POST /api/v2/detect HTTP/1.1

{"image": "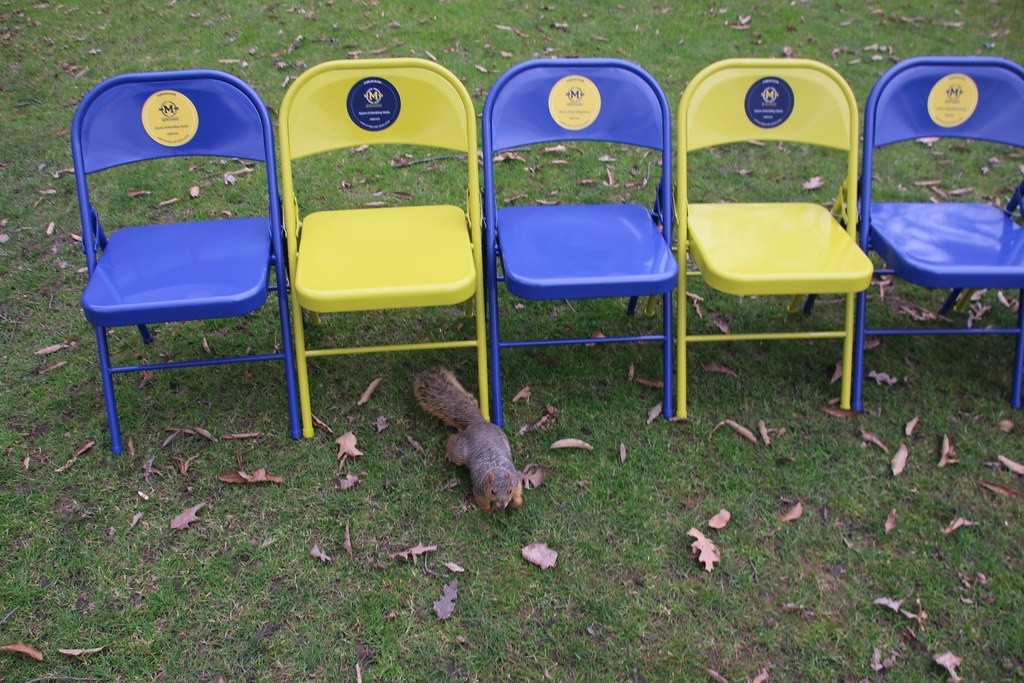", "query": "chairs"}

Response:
[71,70,299,453]
[278,56,492,440]
[670,58,874,420]
[479,57,680,430]
[803,55,1024,412]
